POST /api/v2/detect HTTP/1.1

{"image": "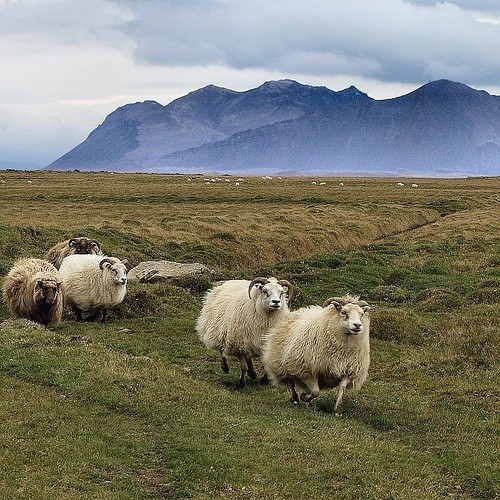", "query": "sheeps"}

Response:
[46,237,101,271]
[262,295,371,418]
[195,276,293,388]
[58,254,129,323]
[3,257,62,323]
